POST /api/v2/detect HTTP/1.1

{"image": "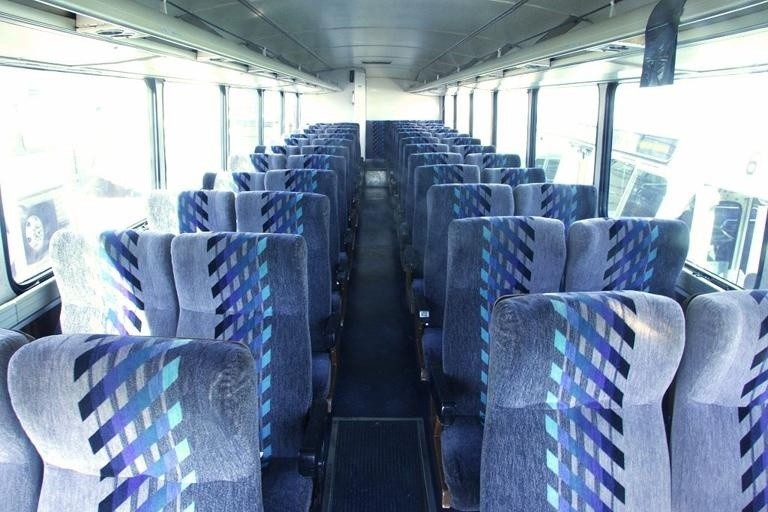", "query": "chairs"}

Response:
[48,227,332,512]
[479,289,686,512]
[671,289,768,512]
[0,327,43,512]
[148,121,363,416]
[430,215,565,511]
[389,120,597,381]
[5,334,265,512]
[563,217,690,298]
[365,120,392,173]
